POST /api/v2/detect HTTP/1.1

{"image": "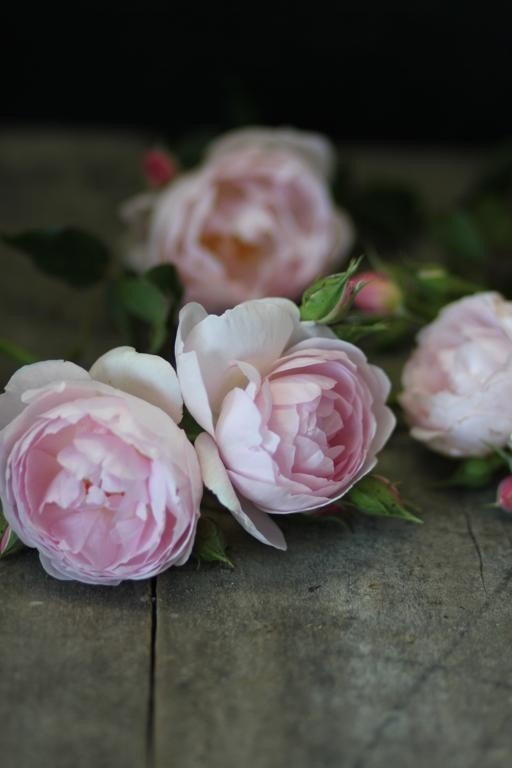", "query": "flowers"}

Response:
[0,115,510,595]
